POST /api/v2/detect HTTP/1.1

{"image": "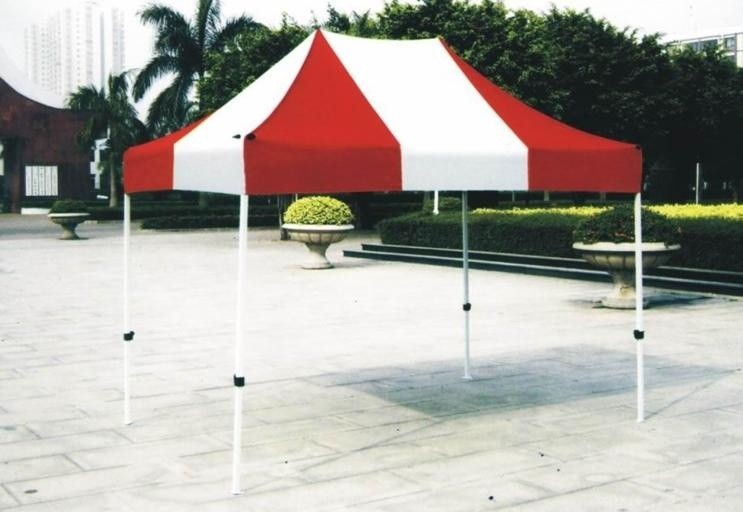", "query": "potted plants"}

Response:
[571,204,683,312]
[279,196,354,270]
[48,200,90,240]
